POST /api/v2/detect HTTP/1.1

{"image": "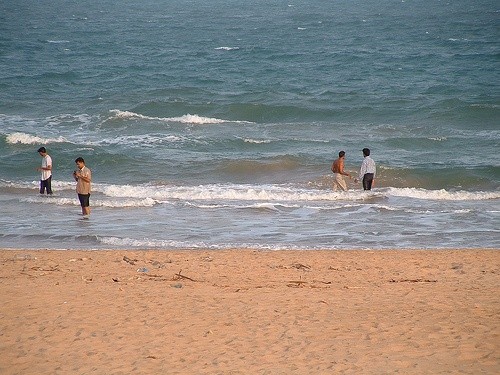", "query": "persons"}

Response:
[73,157,92,216]
[331,151,351,191]
[354,148,377,191]
[37,147,53,195]
